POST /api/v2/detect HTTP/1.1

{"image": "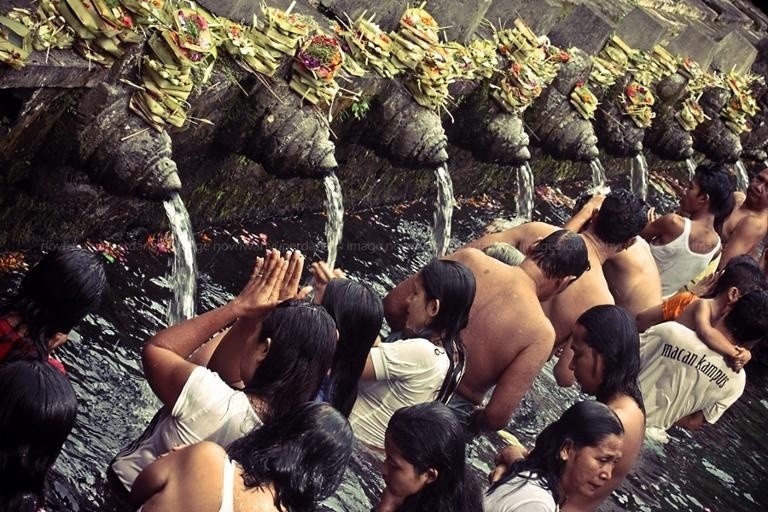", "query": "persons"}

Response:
[110,228,646,509]
[465,166,768,424]
[0,360,100,511]
[0,248,109,375]
